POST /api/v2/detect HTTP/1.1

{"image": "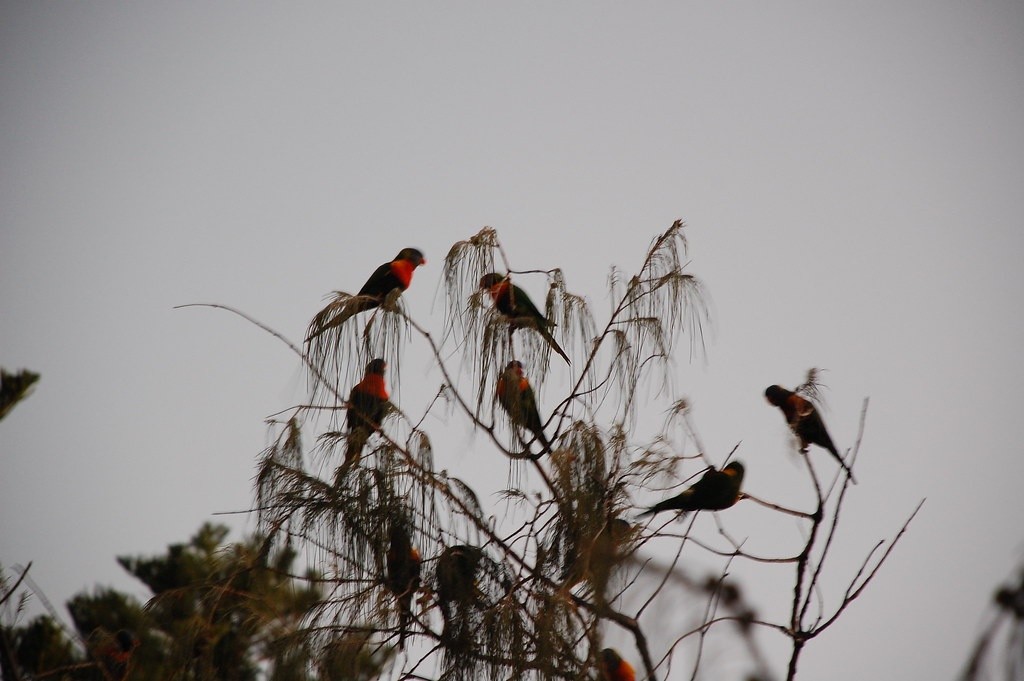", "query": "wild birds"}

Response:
[764,384,859,486]
[595,648,636,681]
[331,358,390,495]
[384,526,423,656]
[477,272,573,368]
[634,461,745,521]
[494,360,556,457]
[301,248,428,344]
